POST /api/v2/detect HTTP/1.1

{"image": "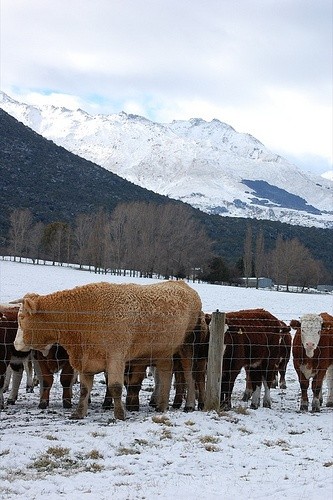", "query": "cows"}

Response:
[289,311,332,413]
[0,279,292,421]
